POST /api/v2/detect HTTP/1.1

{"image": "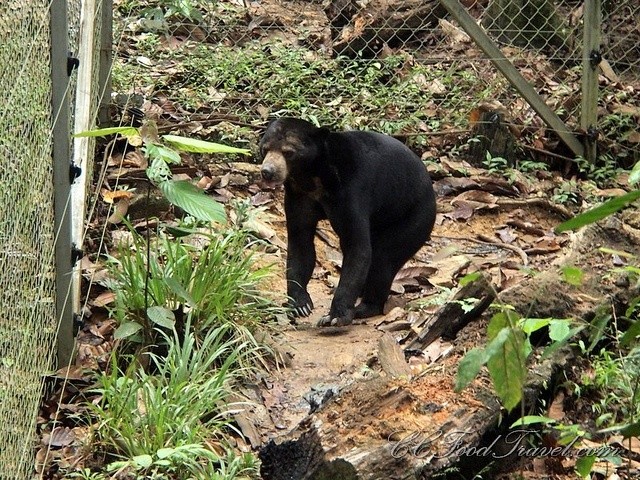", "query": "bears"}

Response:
[259,115,437,328]
